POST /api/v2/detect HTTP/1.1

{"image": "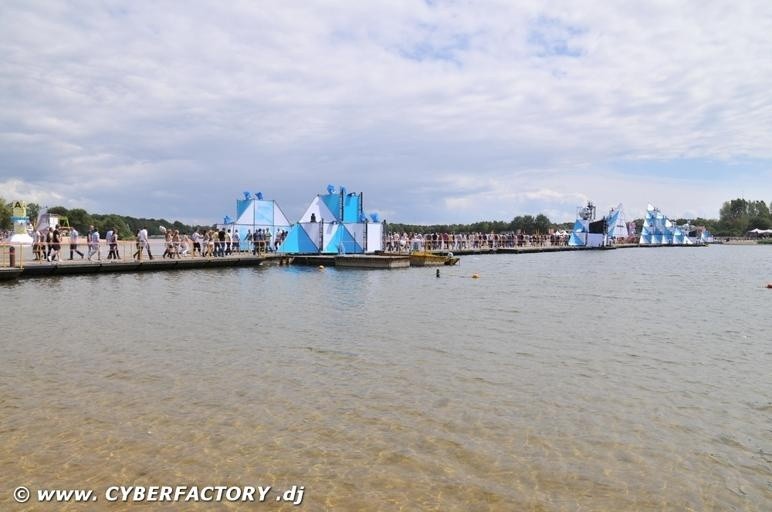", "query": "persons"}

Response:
[310,213,317,223]
[31,224,64,262]
[133,225,155,261]
[162,227,288,259]
[106,227,122,260]
[87,225,103,261]
[610,233,639,246]
[67,226,84,260]
[383,227,569,259]
